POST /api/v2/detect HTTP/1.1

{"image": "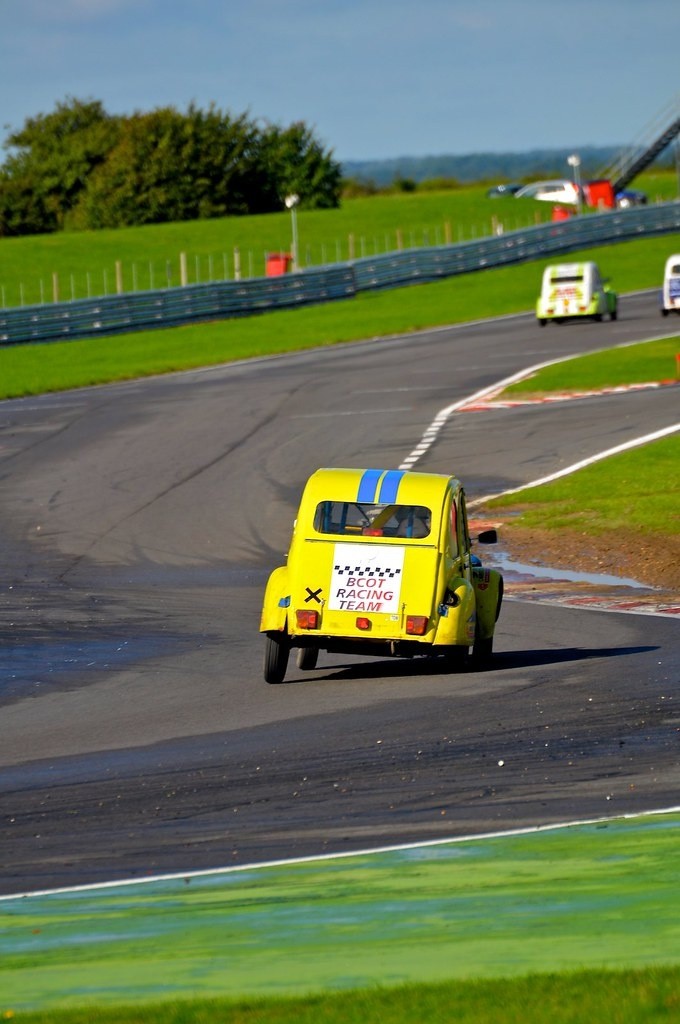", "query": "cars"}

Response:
[513,179,585,209]
[485,181,527,203]
[614,189,645,208]
[257,466,507,686]
[535,259,622,321]
[659,255,680,316]
